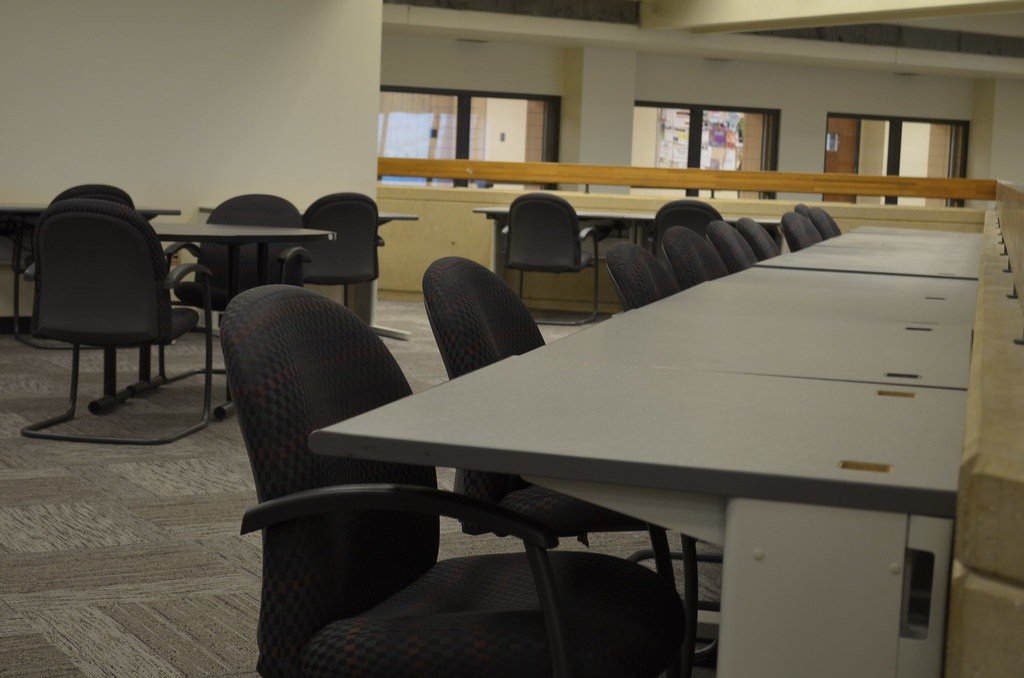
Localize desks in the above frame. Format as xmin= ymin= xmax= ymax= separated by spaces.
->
xmin=91 ymin=221 xmax=338 ymax=420
xmin=0 ymin=196 xmax=184 ymax=223
xmin=316 ymin=231 xmax=984 ymax=678
xmin=199 ymin=203 xmax=421 ymax=345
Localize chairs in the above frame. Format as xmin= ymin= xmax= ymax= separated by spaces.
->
xmin=497 ymin=191 xmax=602 ymax=327
xmin=652 ymin=198 xmax=725 ymax=260
xmin=736 ymin=215 xmax=780 ymax=259
xmin=708 ymin=220 xmax=758 ymax=269
xmin=662 ymin=223 xmax=729 ymax=285
xmin=420 ymin=251 xmax=722 ymax=660
xmin=778 ymin=211 xmax=823 ymax=251
xmin=300 ymin=189 xmax=394 ymax=290
xmin=163 ymin=190 xmax=317 ymax=403
xmin=20 ymin=198 xmax=216 ymax=452
xmin=791 ymin=202 xmax=811 ymax=218
xmin=598 ymin=239 xmax=679 ymax=309
xmin=812 ymin=206 xmax=842 ymax=240
xmin=19 ymin=182 xmax=137 ymax=354
xmin=213 ymin=280 xmax=698 ymax=678
